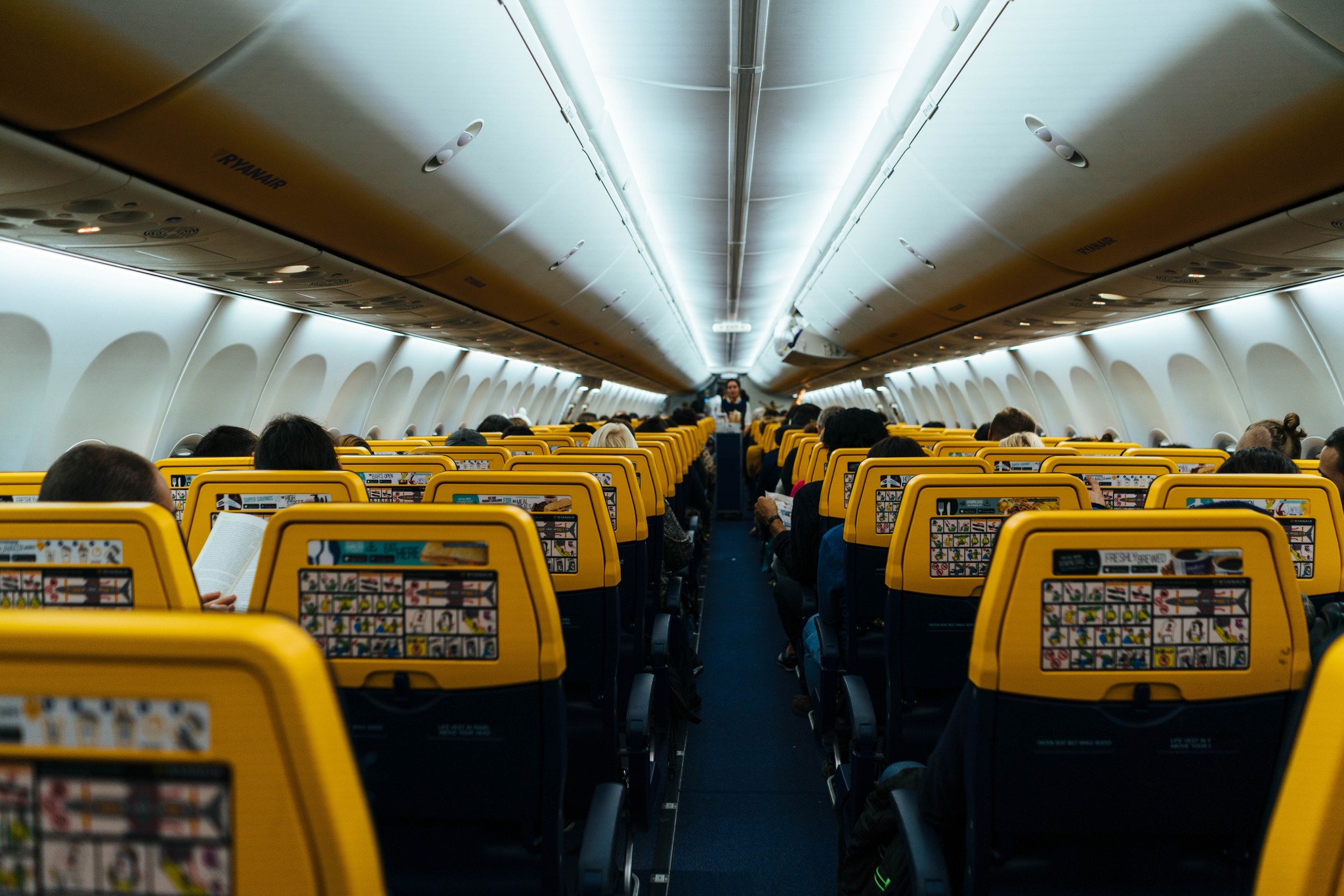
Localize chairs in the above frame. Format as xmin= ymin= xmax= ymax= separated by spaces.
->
xmin=0 ymin=409 xmax=1344 ymax=896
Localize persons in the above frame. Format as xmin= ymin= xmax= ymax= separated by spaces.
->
xmin=37 ymin=444 xmax=237 ymax=611
xmin=720 ymin=379 xmax=752 ymax=438
xmin=194 ymin=425 xmax=259 ymax=457
xmin=444 ymin=428 xmax=488 ymax=446
xmin=335 ymin=434 xmax=372 ymax=455
xmin=1215 ymin=413 xmax=1344 ymax=512
xmin=755 ymin=400 xmax=1046 ymax=668
xmin=254 ymin=412 xmax=343 ymax=471
xmin=476 ymin=398 xmax=717 ymax=571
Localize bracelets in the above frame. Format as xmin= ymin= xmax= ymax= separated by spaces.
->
xmin=767 ymin=515 xmax=784 ymax=528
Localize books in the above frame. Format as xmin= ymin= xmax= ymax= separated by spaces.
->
xmin=192 ymin=512 xmax=268 ymax=612
